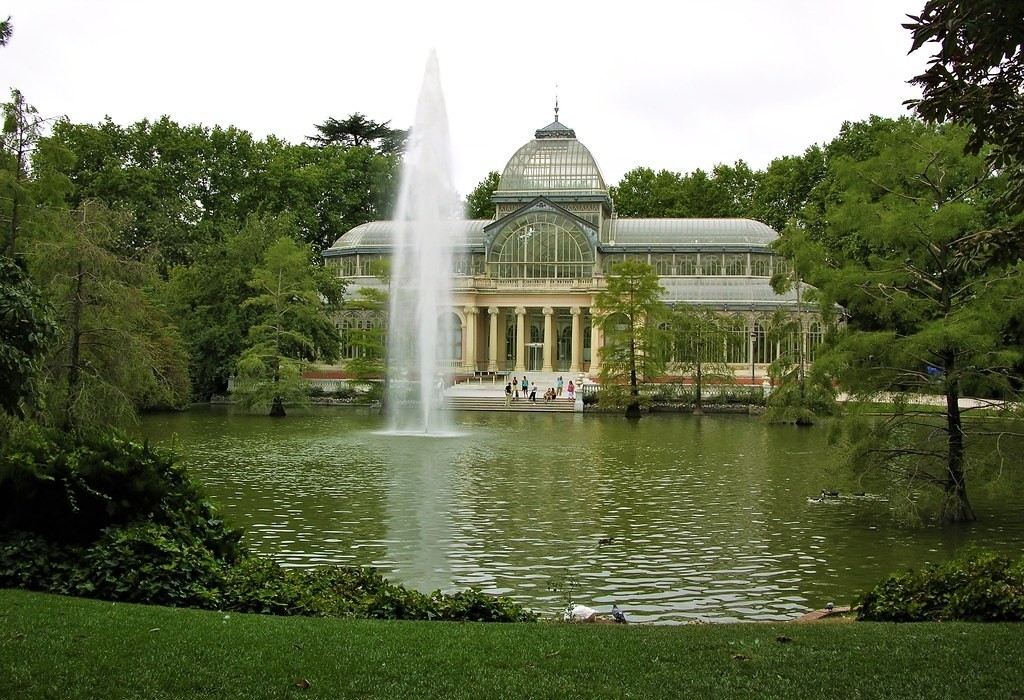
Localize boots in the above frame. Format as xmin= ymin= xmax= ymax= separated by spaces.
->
xmin=514 ymin=397 xmax=518 ymax=401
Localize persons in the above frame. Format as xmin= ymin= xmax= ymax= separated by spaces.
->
xmin=511 ymin=377 xmax=519 ymax=398
xmin=526 ymin=382 xmax=537 ymax=405
xmin=543 ymin=375 xmax=563 ymax=403
xmin=521 ymin=375 xmax=529 ymax=397
xmin=504 ymin=382 xmax=512 ymax=407
xmin=514 ymin=389 xmax=519 ymax=400
xmin=437 ymin=378 xmax=446 ymax=409
xmin=567 ymin=381 xmax=574 ymax=401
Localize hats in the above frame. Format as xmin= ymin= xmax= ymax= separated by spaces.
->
xmin=523 ymin=376 xmax=527 ymax=378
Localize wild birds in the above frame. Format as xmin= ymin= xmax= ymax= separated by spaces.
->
xmin=805 ymin=488 xmax=867 ymax=504
xmin=777 ymin=636 xmax=792 ymax=644
xmin=732 ymin=654 xmax=752 ymax=661
xmin=611 ymin=603 xmax=626 ymax=624
xmin=825 ymin=601 xmax=836 ymax=612
xmin=598 ymin=537 xmax=614 ymax=543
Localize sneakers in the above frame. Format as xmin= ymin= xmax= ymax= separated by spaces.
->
xmin=532 ymin=404 xmax=537 ymax=405
xmin=525 ymin=400 xmax=529 ymax=402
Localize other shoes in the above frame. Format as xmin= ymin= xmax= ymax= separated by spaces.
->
xmin=504 ymin=405 xmax=512 ymax=408
